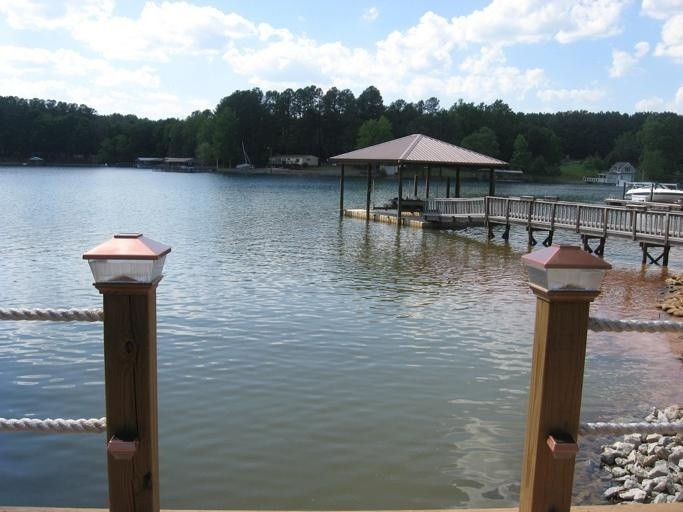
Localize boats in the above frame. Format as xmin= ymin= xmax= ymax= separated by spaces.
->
xmin=624 ymin=188 xmax=683 ymax=203
